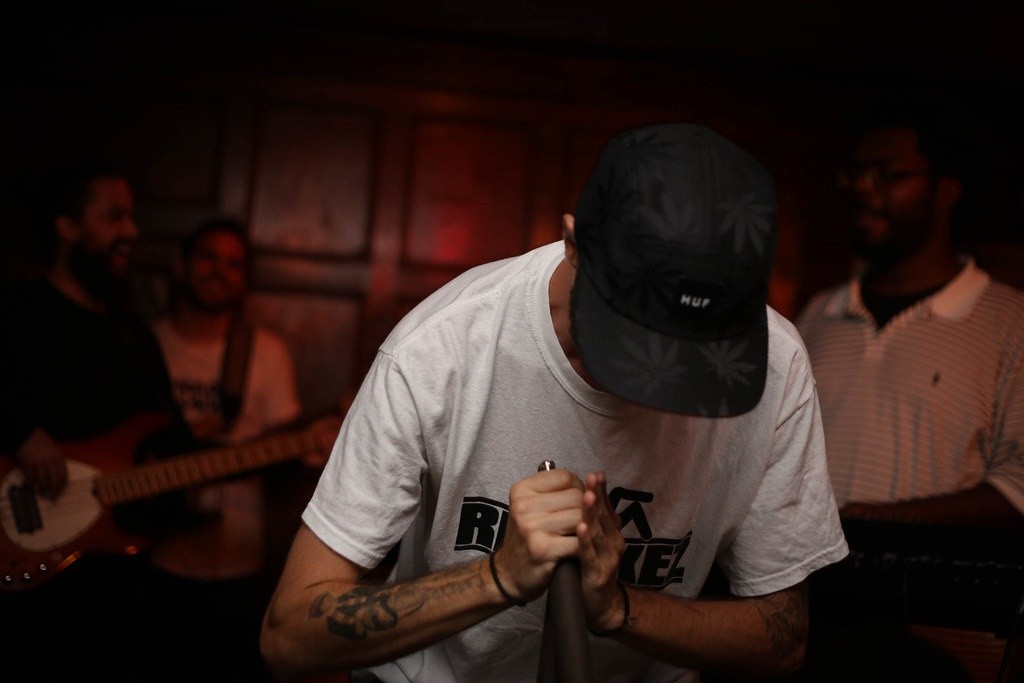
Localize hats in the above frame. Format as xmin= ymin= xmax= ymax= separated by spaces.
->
xmin=570 ymin=122 xmax=783 ymax=420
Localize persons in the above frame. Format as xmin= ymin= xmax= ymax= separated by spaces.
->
xmin=0 ymin=157 xmax=226 ymax=683
xmin=144 ymin=213 xmax=307 ymax=682
xmin=255 ymin=126 xmax=850 ymax=683
xmin=793 ymin=111 xmax=1024 ymax=683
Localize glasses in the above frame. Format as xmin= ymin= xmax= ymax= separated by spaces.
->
xmin=849 ymin=162 xmax=930 ymax=184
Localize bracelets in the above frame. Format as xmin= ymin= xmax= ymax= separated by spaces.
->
xmin=587 ymin=580 xmax=630 ymax=640
xmin=487 ymin=549 xmax=528 ymax=609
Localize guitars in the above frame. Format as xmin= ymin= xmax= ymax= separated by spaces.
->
xmin=0 ymin=403 xmax=351 ymax=593
